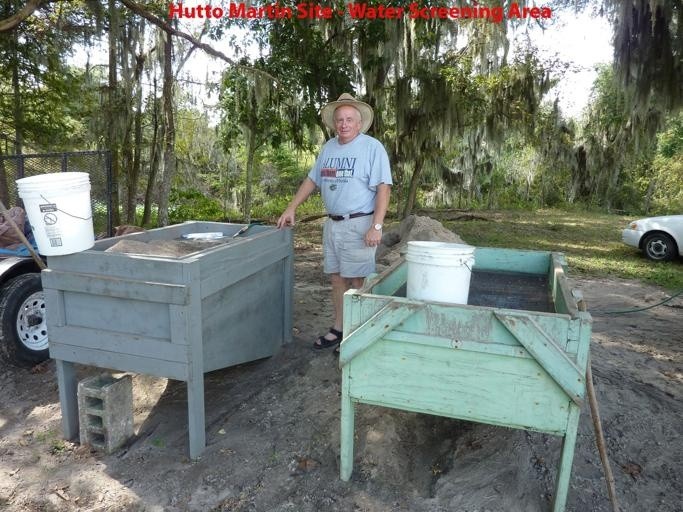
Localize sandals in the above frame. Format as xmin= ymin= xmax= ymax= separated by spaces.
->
xmin=313 ymin=329 xmax=343 ymax=349
xmin=333 ymin=345 xmax=340 ymax=355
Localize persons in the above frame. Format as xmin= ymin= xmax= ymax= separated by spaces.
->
xmin=275 ymin=92 xmax=396 ymax=358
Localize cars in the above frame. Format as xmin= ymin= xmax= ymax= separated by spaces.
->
xmin=0 ymin=248 xmax=51 ymax=368
xmin=621 ymin=213 xmax=683 ymax=262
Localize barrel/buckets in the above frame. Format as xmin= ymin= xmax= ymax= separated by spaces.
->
xmin=14 ymin=170 xmax=96 ymax=257
xmin=404 ymin=241 xmax=476 ymax=306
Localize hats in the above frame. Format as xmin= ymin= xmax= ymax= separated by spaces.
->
xmin=321 ymin=93 xmax=374 ymax=134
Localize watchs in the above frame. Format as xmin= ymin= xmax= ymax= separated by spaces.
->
xmin=371 ymin=222 xmax=383 ymax=230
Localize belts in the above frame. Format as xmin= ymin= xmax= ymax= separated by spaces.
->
xmin=328 ymin=211 xmax=374 ymax=221
